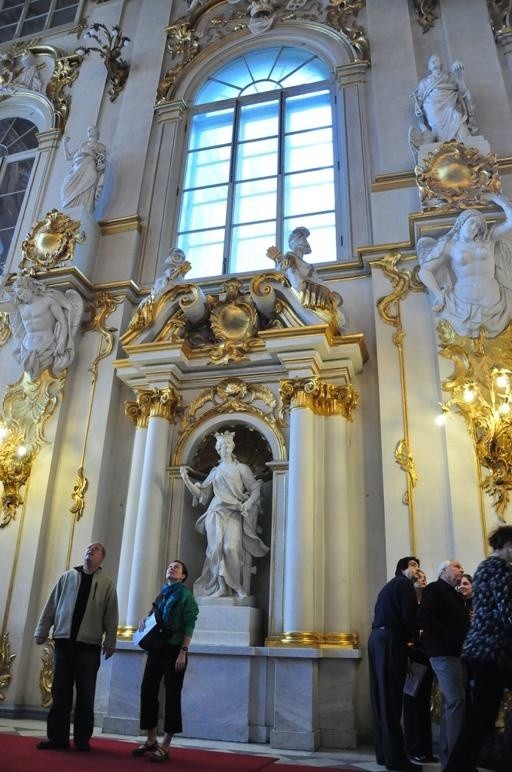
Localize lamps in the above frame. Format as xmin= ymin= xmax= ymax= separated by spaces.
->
xmin=0 ymin=429 xmax=36 ymax=530
xmin=75 ymin=23 xmax=132 ymax=103
xmin=460 ymin=363 xmax=512 ymax=524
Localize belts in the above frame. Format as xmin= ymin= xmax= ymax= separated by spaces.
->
xmin=372 ymin=625 xmax=397 ymax=631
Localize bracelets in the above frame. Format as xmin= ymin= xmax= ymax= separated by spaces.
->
xmin=179 ymin=645 xmax=189 ymax=652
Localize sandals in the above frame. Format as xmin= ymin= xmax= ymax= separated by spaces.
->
xmin=149 ymin=746 xmax=172 ymax=762
xmin=129 ymin=738 xmax=160 ymax=757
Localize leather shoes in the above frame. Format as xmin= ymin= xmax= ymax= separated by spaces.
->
xmin=35 ymin=735 xmax=92 ymax=751
xmin=408 ymin=747 xmax=438 ymax=764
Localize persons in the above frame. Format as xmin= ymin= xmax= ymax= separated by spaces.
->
xmin=131 ymin=560 xmax=198 ymax=762
xmin=32 ymin=540 xmax=119 ymax=753
xmin=368 ymin=525 xmax=512 ymax=772
xmin=8 ymin=275 xmax=82 ymax=381
xmin=57 ymin=125 xmax=111 ymax=215
xmin=408 ymin=50 xmax=479 ymax=143
xmin=177 ymin=427 xmax=266 ymax=601
xmin=415 ymin=193 xmax=511 ymax=338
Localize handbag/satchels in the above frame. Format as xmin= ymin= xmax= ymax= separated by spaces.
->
xmin=139 ymin=605 xmax=174 ymax=655
